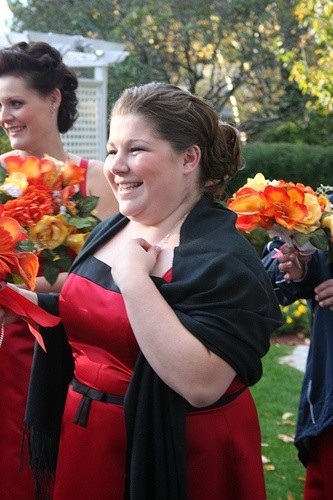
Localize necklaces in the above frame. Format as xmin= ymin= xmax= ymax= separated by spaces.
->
xmin=16 ymin=150 xmax=20 ymax=157
xmin=158 ymin=212 xmax=190 ymax=247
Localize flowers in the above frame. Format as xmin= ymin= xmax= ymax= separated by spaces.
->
xmin=226 ymin=173 xmax=333 ymax=269
xmin=0 ymin=152 xmax=103 ymax=292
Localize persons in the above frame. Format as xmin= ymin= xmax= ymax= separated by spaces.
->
xmin=261 ymin=194 xmax=333 ymax=500
xmin=0 ymin=81 xmax=285 ymax=500
xmin=0 ymin=41 xmax=119 ymax=500
